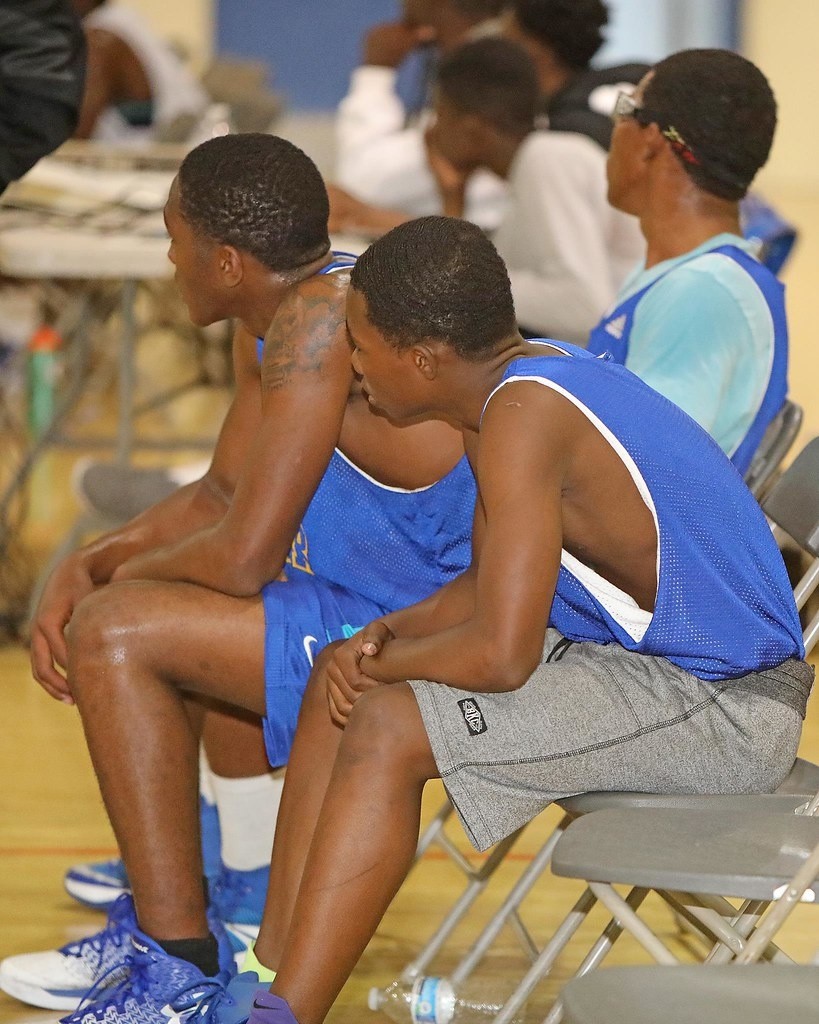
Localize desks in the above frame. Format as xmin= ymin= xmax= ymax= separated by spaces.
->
xmin=0 ymin=135 xmax=189 ymax=646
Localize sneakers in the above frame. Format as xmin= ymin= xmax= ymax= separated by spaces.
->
xmin=65 ymin=792 xmax=271 ymax=939
xmin=58 ymin=929 xmax=238 ymax=1024
xmin=170 ymin=971 xmax=275 ymax=1024
xmin=0 ymin=892 xmax=238 ymax=1012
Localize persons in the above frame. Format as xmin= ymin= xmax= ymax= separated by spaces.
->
xmin=165 ymin=213 xmax=816 ymax=1024
xmin=425 ymin=33 xmax=648 ymax=341
xmin=1 ymin=0 xmax=652 ymax=256
xmin=0 ymin=129 xmax=481 ymax=1024
xmin=65 ymin=44 xmax=794 ymax=955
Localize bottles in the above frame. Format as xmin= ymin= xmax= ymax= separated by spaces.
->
xmin=369 ymin=974 xmax=527 ymax=1024
xmin=25 ymin=324 xmax=64 ymax=443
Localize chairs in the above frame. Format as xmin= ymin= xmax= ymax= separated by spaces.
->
xmin=57 ymin=60 xmax=286 ymax=376
xmin=397 ymin=395 xmax=819 ymax=1024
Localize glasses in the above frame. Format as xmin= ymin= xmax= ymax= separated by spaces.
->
xmin=613 ymin=91 xmax=649 ymax=126
